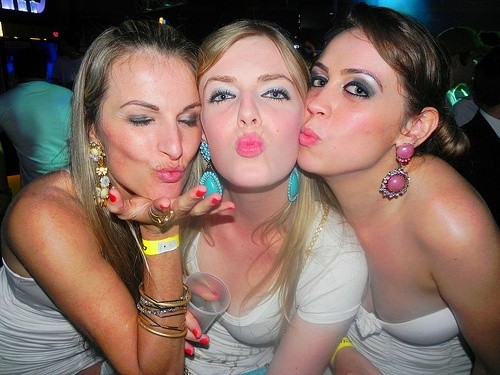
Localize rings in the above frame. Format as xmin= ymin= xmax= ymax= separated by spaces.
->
xmin=149 ymin=207 xmax=174 ymax=226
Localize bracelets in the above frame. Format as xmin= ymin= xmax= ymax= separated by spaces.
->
xmin=142 ymin=234 xmax=179 ymax=256
xmin=329 ymin=338 xmax=353 ymax=369
xmin=137 ymin=282 xmax=190 ymax=338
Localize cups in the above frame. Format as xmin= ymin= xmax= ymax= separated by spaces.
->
xmin=184 ymin=271 xmax=231 ymax=332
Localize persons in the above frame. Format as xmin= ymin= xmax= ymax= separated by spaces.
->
xmin=177 ymin=21 xmax=369 ymax=375
xmin=436 ymin=27 xmax=500 ymax=232
xmin=296 ymin=0 xmax=500 ymax=375
xmin=0 ymin=40 xmax=74 ymax=188
xmin=0 ymin=18 xmax=235 ymax=375
